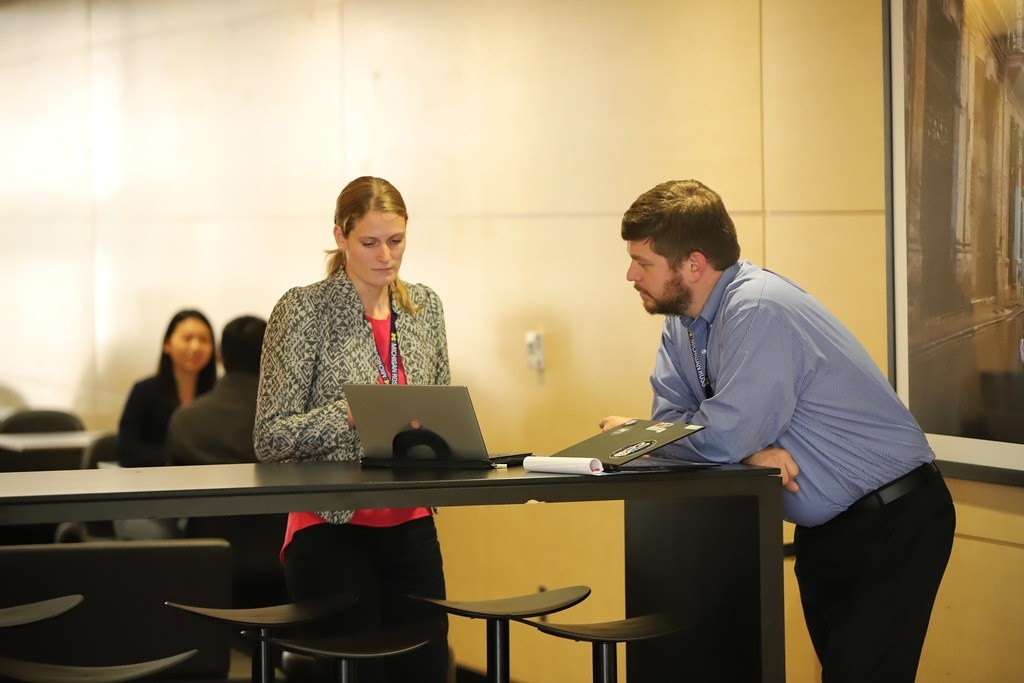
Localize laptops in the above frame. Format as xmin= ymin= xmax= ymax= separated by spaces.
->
xmin=547 ymin=419 xmax=723 ymax=473
xmin=342 ymin=383 xmax=534 ymax=462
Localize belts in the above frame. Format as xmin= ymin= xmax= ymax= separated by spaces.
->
xmin=822 ymin=461 xmax=939 ymax=528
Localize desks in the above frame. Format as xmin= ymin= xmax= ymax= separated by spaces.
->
xmin=0 ymin=427 xmax=118 ymax=468
xmin=0 ymin=463 xmax=786 ymax=683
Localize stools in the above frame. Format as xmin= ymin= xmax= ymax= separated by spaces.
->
xmin=0 ymin=586 xmax=693 ymax=683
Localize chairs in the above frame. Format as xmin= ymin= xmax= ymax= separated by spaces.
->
xmin=0 ymin=410 xmax=86 ymax=472
xmin=79 ymin=434 xmax=121 ymax=539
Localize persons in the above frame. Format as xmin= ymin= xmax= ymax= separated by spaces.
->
xmin=164 ymin=317 xmax=292 ymax=606
xmin=597 ymin=180 xmax=958 ymax=683
xmin=252 ymin=176 xmax=459 ymax=683
xmin=117 ymin=310 xmax=248 ymax=539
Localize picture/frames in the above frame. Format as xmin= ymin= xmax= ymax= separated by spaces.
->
xmin=880 ymin=0 xmax=1024 ymax=487
